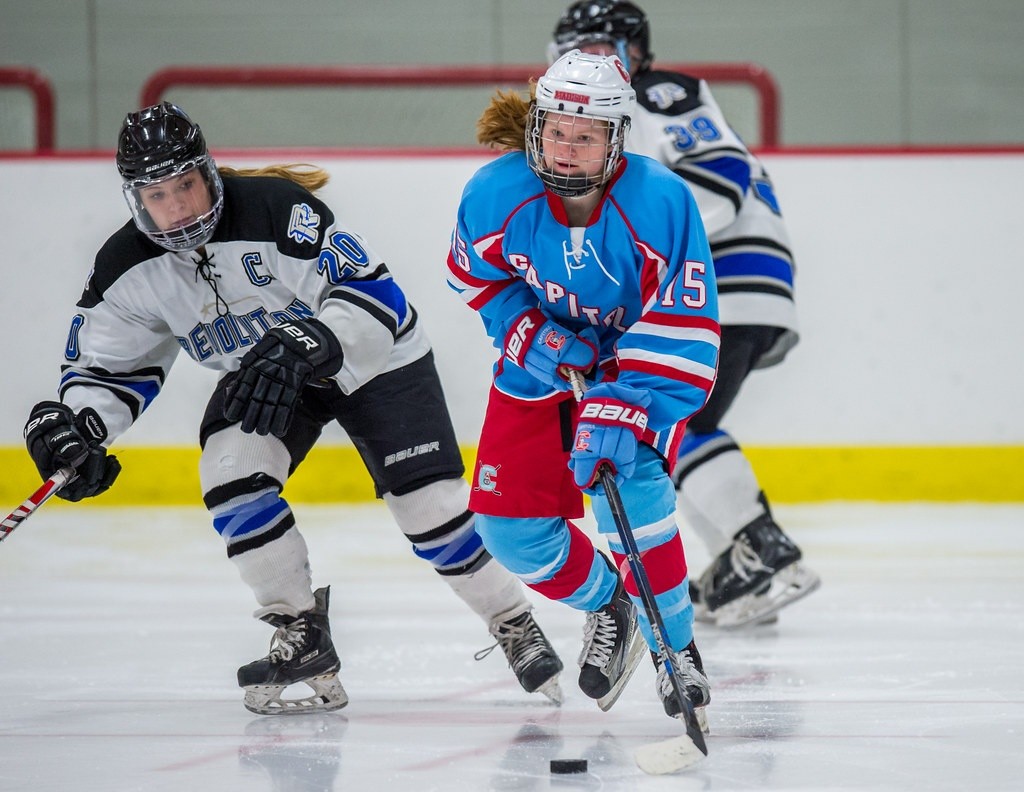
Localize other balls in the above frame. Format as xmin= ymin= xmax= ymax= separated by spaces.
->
xmin=550 ymin=758 xmax=588 ymax=774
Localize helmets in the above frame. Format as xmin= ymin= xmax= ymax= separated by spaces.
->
xmin=545 ymin=0 xmax=653 ymax=88
xmin=524 ymin=48 xmax=636 ymax=199
xmin=114 ymin=101 xmax=223 ymax=252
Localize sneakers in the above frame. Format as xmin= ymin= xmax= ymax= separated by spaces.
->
xmin=707 ymin=489 xmax=821 ymax=625
xmin=576 ymin=550 xmax=649 ymax=711
xmin=650 ymin=636 xmax=711 ymax=737
xmin=237 ymin=585 xmax=349 ymax=714
xmin=473 ymin=602 xmax=563 ymax=706
xmin=686 ymin=547 xmax=778 ymax=626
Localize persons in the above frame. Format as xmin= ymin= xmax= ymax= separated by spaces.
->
xmin=25 ymin=102 xmax=563 ymax=715
xmin=448 ymin=49 xmax=711 ymax=732
xmin=550 ymin=0 xmax=822 ymax=629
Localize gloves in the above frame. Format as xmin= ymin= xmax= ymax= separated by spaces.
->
xmin=493 ymin=306 xmax=598 ymax=392
xmin=24 ymin=401 xmax=122 ymax=503
xmin=568 ymin=382 xmax=652 ymax=496
xmin=223 ymin=316 xmax=344 ymax=439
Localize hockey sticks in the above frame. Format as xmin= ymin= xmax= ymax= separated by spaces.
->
xmin=0 ymin=462 xmax=85 ymax=541
xmin=571 ymin=365 xmax=710 ymax=777
